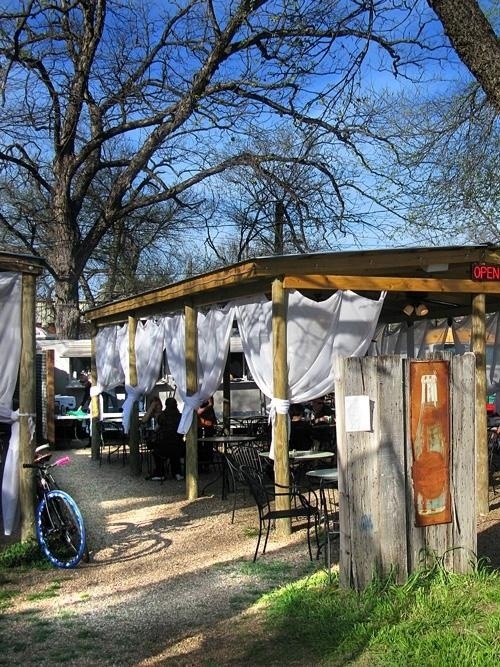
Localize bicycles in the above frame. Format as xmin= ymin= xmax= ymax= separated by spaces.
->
xmin=23 ymin=451 xmax=89 ymax=568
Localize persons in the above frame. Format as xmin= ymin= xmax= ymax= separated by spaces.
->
xmin=77 ymin=375 xmax=92 ymax=449
xmin=139 ymin=396 xmax=217 ymax=482
xmin=290 ymin=399 xmax=336 ymax=486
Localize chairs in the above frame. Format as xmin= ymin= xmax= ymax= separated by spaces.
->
xmin=95 ymin=415 xmax=342 ymax=574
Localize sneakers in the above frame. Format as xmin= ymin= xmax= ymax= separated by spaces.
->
xmin=152 ymin=474 xmax=165 ymax=480
xmin=176 ymin=473 xmax=184 ymax=480
